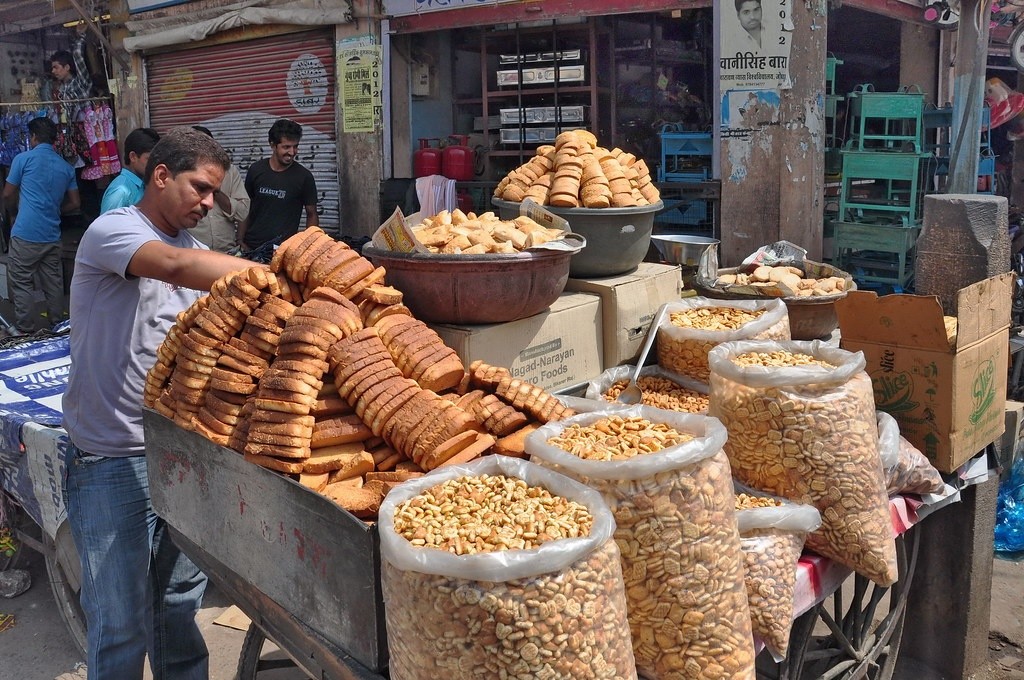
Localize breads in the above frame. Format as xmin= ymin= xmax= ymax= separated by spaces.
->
xmin=718 ymin=265 xmax=844 ymax=298
xmin=141 ymin=226 xmax=579 ymax=520
xmin=407 ymin=208 xmax=566 ymax=256
xmin=492 ymin=129 xmax=661 ymax=208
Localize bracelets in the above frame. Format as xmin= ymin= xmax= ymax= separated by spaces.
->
xmin=237 ymin=239 xmax=244 ymax=241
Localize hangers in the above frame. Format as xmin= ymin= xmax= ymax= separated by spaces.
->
xmin=5 ymin=96 xmax=107 ymax=118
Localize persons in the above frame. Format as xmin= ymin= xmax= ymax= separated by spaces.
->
xmin=724 ymin=0 xmax=771 ymax=49
xmin=237 ymin=119 xmax=319 ymax=252
xmin=100 ymin=128 xmax=160 ymax=215
xmin=50 ymin=20 xmax=92 ymax=169
xmin=61 ymin=126 xmax=272 ymax=680
xmin=185 ymin=125 xmax=251 ymax=251
xmin=0 ymin=117 xmax=78 ymax=333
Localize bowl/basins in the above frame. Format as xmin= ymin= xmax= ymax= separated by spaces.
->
xmin=362 ymin=230 xmax=572 ymax=326
xmin=490 ymin=195 xmax=662 ymax=276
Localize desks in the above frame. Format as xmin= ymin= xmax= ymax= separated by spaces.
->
xmin=658 ymin=132 xmax=713 ymax=181
xmin=823 ymin=49 xmax=997 ymax=294
xmin=654 ymin=165 xmax=707 ymax=225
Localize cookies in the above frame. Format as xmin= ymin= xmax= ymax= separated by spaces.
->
xmin=379 ymin=306 xmax=945 ymax=680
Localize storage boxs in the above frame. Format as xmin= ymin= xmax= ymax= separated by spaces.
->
xmin=426 ymin=289 xmax=603 ymax=393
xmin=835 ymin=272 xmax=1016 ymax=476
xmin=561 ymin=261 xmax=683 ymax=372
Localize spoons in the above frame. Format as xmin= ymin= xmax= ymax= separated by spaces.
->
xmin=616 ymin=302 xmax=667 ymax=406
